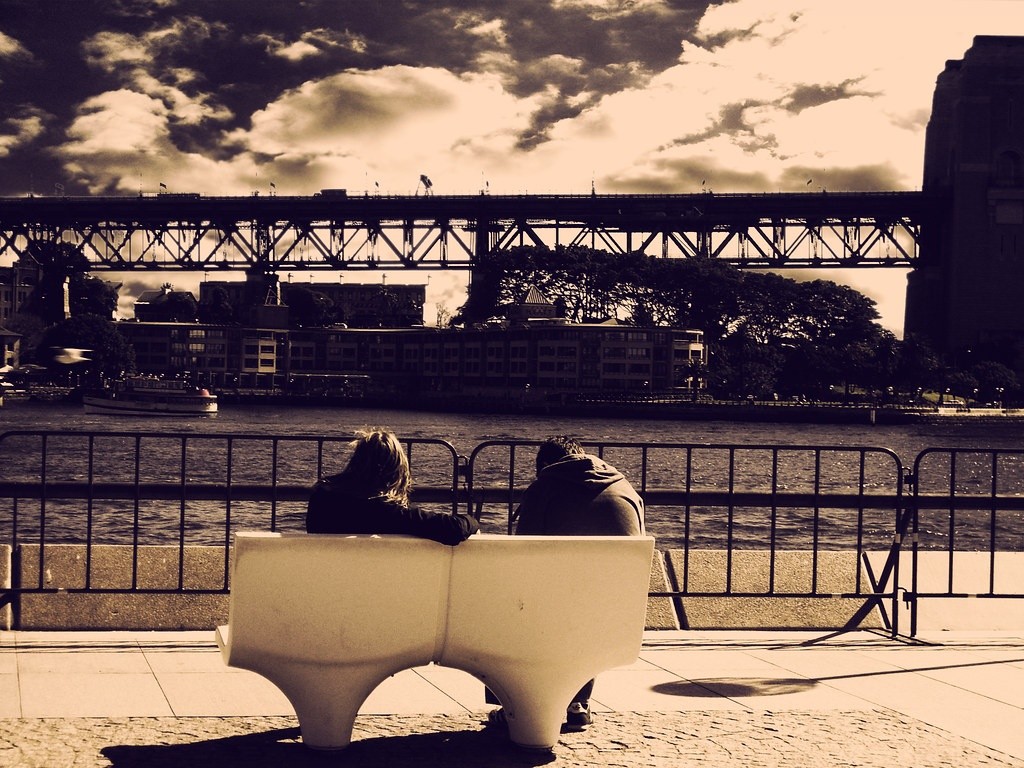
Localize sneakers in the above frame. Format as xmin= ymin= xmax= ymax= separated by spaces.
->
xmin=488 ymin=707 xmax=508 ymax=729
xmin=567 ymin=702 xmax=593 ymax=724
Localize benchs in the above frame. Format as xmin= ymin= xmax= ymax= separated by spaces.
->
xmin=214 ymin=530 xmax=656 ymax=752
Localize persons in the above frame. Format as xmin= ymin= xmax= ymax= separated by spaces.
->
xmin=480 ymin=434 xmax=646 ymax=732
xmin=306 ymin=425 xmax=480 ymax=546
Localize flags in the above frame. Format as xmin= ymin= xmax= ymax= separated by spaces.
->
xmin=270 ymin=182 xmax=275 ymax=188
xmin=420 ymin=174 xmax=433 ymax=189
xmin=160 ymin=183 xmax=166 ymax=188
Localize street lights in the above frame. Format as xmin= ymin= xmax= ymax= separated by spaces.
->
xmin=916 ymin=387 xmax=922 ymax=407
xmin=996 ymin=387 xmax=1004 ymax=408
xmin=946 ymin=387 xmax=951 ymax=407
xmin=888 ymin=386 xmax=894 ymax=404
xmin=974 ymin=388 xmax=980 ymax=408
xmin=643 ymin=380 xmax=648 ymax=397
xmin=829 ymin=384 xmax=834 ymax=402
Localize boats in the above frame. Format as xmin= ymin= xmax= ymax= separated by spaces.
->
xmin=82 ymin=371 xmax=218 ymax=418
